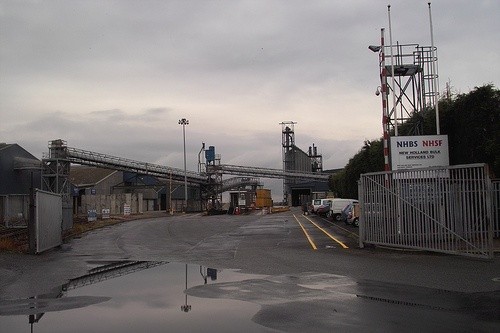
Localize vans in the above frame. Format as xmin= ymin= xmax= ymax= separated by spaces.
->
xmin=311 ymin=200 xmax=332 ymax=216
xmin=329 ymin=200 xmax=359 ymax=222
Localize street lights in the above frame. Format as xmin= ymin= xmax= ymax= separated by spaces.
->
xmin=178 ymin=118 xmax=189 ymax=215
xmin=180 ymin=265 xmax=191 ymax=312
xmin=368 ymin=28 xmax=390 ymax=190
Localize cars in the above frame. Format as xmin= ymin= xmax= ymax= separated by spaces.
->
xmin=347 ymin=204 xmax=384 ymax=228
xmin=341 ymin=202 xmax=359 ymax=225
xmin=317 ymin=204 xmax=329 ymax=219
